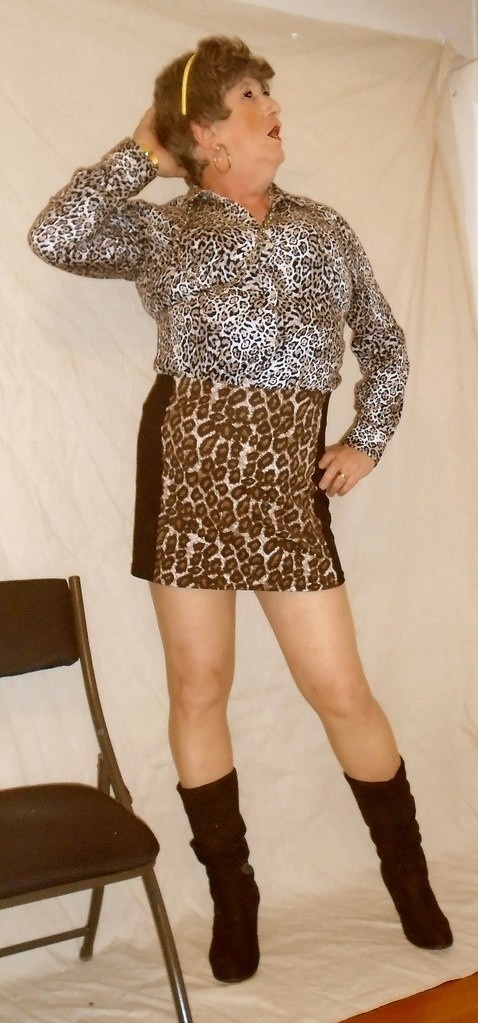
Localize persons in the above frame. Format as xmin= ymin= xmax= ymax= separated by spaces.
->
xmin=28 ymin=37 xmax=452 ymax=983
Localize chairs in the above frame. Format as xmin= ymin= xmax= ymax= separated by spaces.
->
xmin=0 ymin=576 xmax=192 ymax=1023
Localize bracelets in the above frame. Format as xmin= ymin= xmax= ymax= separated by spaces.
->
xmin=135 ymin=140 xmax=160 ymax=168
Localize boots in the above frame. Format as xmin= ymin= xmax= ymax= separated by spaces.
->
xmin=175 ymin=765 xmax=261 ymax=982
xmin=344 ymin=753 xmax=454 ymax=950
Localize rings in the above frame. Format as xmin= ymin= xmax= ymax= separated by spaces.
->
xmin=336 ymin=472 xmax=348 ymax=480
